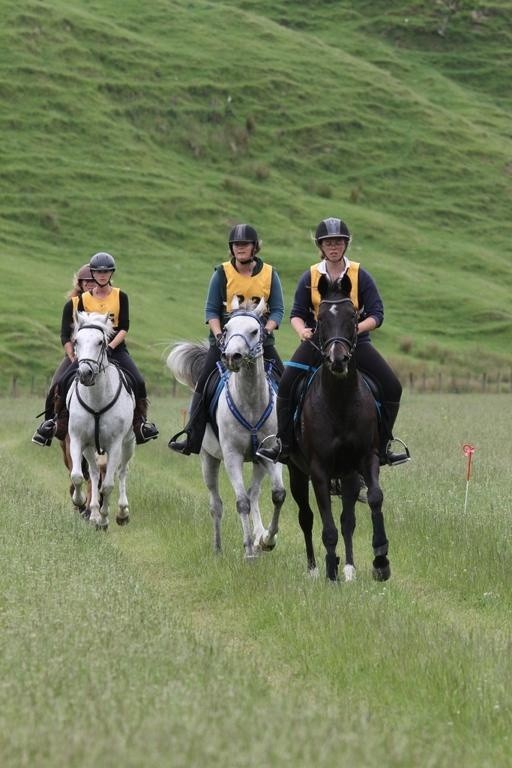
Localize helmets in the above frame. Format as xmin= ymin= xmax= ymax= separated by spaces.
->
xmin=315 ymin=218 xmax=350 ymax=240
xmin=77 ymin=252 xmax=116 ymax=280
xmin=228 ymin=224 xmax=257 ymax=243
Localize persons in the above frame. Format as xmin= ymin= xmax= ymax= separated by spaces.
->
xmin=33 ymin=264 xmax=98 ymax=446
xmin=168 ymin=223 xmax=286 ymax=455
xmin=38 ymin=252 xmax=158 ymax=444
xmin=257 ymin=216 xmax=408 ymax=465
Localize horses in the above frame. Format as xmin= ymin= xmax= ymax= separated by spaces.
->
xmin=286 ymin=271 xmax=394 ymax=585
xmin=56 ymin=312 xmax=140 ymax=532
xmin=162 ymin=293 xmax=288 ymax=561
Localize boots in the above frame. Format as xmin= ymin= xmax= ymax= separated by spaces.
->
xmin=133 ymin=397 xmax=159 ymax=445
xmin=34 ymin=392 xmax=69 ymax=446
xmin=170 ymin=392 xmax=206 ymax=454
xmin=258 ymin=397 xmax=294 ymax=464
xmin=379 ymin=402 xmax=408 ymax=466
